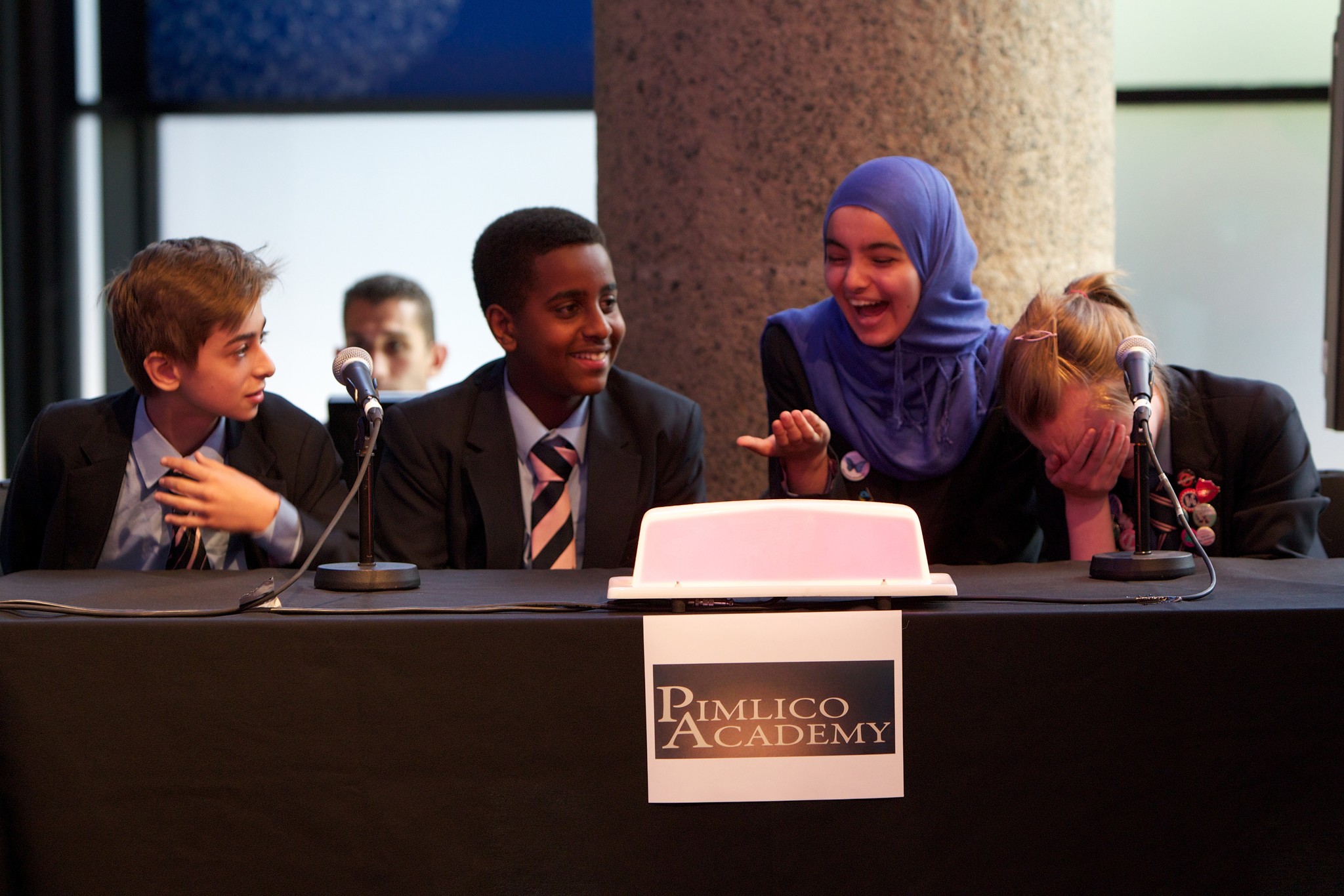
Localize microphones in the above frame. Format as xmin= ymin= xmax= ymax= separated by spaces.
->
xmin=1115 ymin=335 xmax=1156 ymax=422
xmin=333 ymin=348 xmax=385 ymax=421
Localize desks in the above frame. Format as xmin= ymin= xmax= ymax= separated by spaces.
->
xmin=0 ymin=559 xmax=1344 ymax=896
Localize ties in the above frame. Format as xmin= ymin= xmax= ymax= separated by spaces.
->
xmin=1145 ymin=467 xmax=1181 ymax=552
xmin=164 ymin=466 xmax=211 ymax=570
xmin=530 ymin=434 xmax=577 ymax=570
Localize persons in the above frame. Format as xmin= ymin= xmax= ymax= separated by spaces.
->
xmin=372 ymin=206 xmax=704 ymax=569
xmin=738 ymin=157 xmax=1049 ymax=563
xmin=1 ymin=236 xmax=390 ymax=574
xmin=321 ymin=273 xmax=447 ymax=429
xmin=997 ymin=262 xmax=1331 ymax=562
